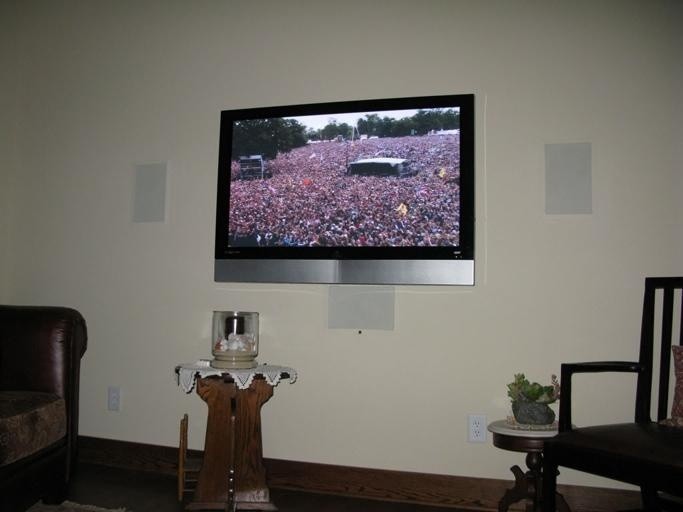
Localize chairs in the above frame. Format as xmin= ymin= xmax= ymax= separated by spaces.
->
xmin=535 ymin=274 xmax=683 ymax=511
xmin=177 ymin=411 xmax=204 ymax=502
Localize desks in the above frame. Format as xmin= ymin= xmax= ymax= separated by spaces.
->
xmin=174 ymin=356 xmax=295 ymax=511
xmin=485 ymin=413 xmax=578 ymax=511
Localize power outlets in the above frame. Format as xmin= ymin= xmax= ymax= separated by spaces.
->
xmin=463 ymin=413 xmax=487 ymax=446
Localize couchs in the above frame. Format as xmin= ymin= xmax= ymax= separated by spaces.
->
xmin=0 ymin=303 xmax=87 ymax=505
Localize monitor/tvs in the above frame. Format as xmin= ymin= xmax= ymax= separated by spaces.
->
xmin=214 ymin=94 xmax=474 ymax=285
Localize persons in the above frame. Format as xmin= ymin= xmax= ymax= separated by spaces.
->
xmin=225 ymin=130 xmax=459 ymax=246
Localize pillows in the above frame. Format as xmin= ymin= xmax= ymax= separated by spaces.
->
xmin=657 ymin=344 xmax=682 ymax=429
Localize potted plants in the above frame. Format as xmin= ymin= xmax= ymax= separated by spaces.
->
xmin=501 ymin=367 xmax=559 ymax=429
